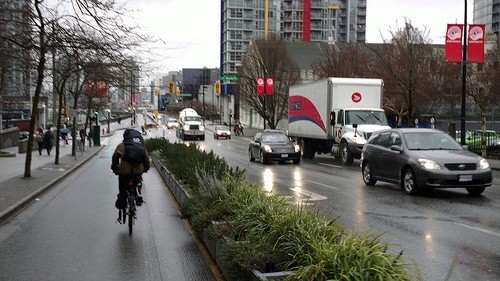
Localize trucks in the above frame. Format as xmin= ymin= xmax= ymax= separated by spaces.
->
xmin=287 ymin=78 xmax=394 ymax=168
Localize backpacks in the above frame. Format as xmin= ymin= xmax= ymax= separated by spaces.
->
xmin=123 ymin=132 xmax=146 ymax=165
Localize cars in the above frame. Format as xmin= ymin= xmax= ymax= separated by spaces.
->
xmin=455 ymin=129 xmax=500 ymax=155
xmin=248 ymin=128 xmax=301 ymax=165
xmin=360 ymin=127 xmax=493 ymax=197
xmin=165 ymin=118 xmax=178 ymax=129
xmin=213 ymin=125 xmax=231 ymax=140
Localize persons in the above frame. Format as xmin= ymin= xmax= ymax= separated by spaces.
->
xmin=44 ymin=126 xmax=55 ymax=154
xmin=110 ymin=129 xmax=150 ymax=224
xmin=233 ymin=119 xmax=243 ymax=135
xmin=60 ymin=132 xmax=67 ymax=139
xmin=35 ymin=130 xmax=44 ymax=155
xmin=80 ymin=128 xmax=87 ymax=142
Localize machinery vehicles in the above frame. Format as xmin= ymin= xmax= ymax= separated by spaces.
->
xmin=175 ymin=107 xmax=205 ymax=141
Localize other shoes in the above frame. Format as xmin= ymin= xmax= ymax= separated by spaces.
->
xmin=136 ymin=196 xmax=143 ymax=206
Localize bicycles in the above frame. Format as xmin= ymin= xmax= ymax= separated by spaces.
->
xmin=111 ymin=166 xmax=147 ymax=235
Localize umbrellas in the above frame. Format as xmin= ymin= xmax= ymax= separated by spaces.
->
xmin=60 ymin=128 xmax=70 ymax=133
